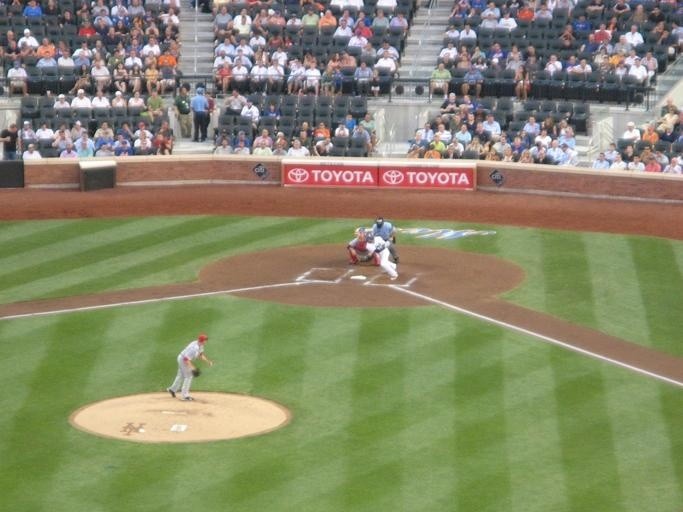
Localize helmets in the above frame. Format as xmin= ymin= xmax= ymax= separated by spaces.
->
xmin=355 ymin=227 xmax=374 ymax=240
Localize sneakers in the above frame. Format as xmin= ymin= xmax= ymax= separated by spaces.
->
xmin=183 ymin=396 xmax=193 ymax=401
xmin=166 ymin=388 xmax=176 ymax=398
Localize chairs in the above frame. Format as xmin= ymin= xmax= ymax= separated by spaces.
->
xmin=2 ymin=0 xmax=682 ymax=163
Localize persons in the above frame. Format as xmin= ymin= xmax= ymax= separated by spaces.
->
xmin=407 ymin=1 xmax=682 ymax=174
xmin=347 ymin=217 xmax=401 ymax=281
xmin=0 ymin=0 xmax=214 ymax=158
xmin=166 ymin=335 xmax=212 ymax=401
xmin=193 ymin=0 xmax=418 ymax=156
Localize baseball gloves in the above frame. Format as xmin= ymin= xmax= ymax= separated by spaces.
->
xmin=359 ymin=255 xmax=373 ymax=262
xmin=192 ymin=368 xmax=201 ymax=376
xmin=374 ymin=244 xmax=385 ymax=252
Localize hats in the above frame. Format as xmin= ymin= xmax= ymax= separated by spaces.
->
xmin=199 ymin=335 xmax=207 ymax=341
xmin=376 ymin=217 xmax=384 ymax=225
